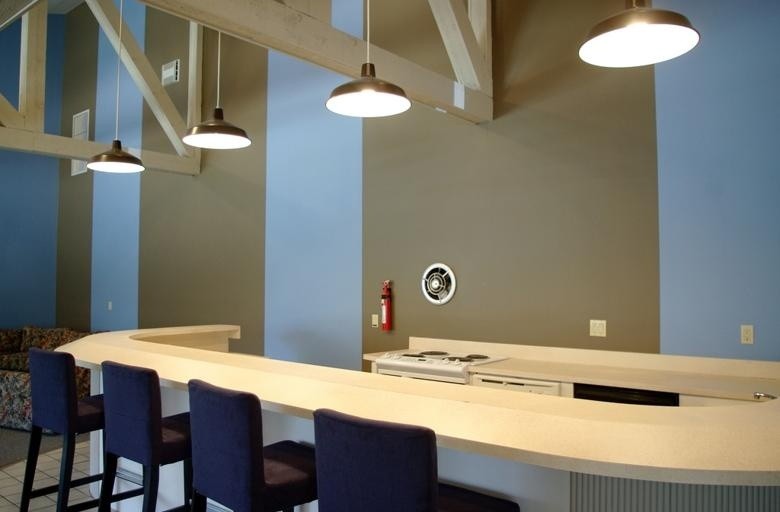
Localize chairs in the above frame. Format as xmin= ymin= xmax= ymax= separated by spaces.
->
xmin=97 ymin=358 xmax=207 ymax=512
xmin=311 ymin=407 xmax=519 ymax=512
xmin=186 ymin=375 xmax=319 ymax=511
xmin=20 ymin=348 xmax=118 ymax=511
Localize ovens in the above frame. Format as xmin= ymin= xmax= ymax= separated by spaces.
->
xmin=377 ymin=363 xmax=466 ymax=384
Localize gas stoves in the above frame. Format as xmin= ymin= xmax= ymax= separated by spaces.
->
xmin=380 ymin=349 xmax=504 ymax=370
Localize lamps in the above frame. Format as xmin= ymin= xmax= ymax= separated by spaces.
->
xmin=85 ymin=2 xmax=146 ymax=177
xmin=325 ymin=1 xmax=412 ymax=122
xmin=183 ymin=28 xmax=252 ymax=152
xmin=578 ymin=0 xmax=701 ymax=73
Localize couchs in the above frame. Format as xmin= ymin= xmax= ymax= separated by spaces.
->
xmin=0 ymin=323 xmax=108 ymax=434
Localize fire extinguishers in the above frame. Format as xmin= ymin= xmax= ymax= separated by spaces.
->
xmin=380 ymin=280 xmax=392 ymax=331
